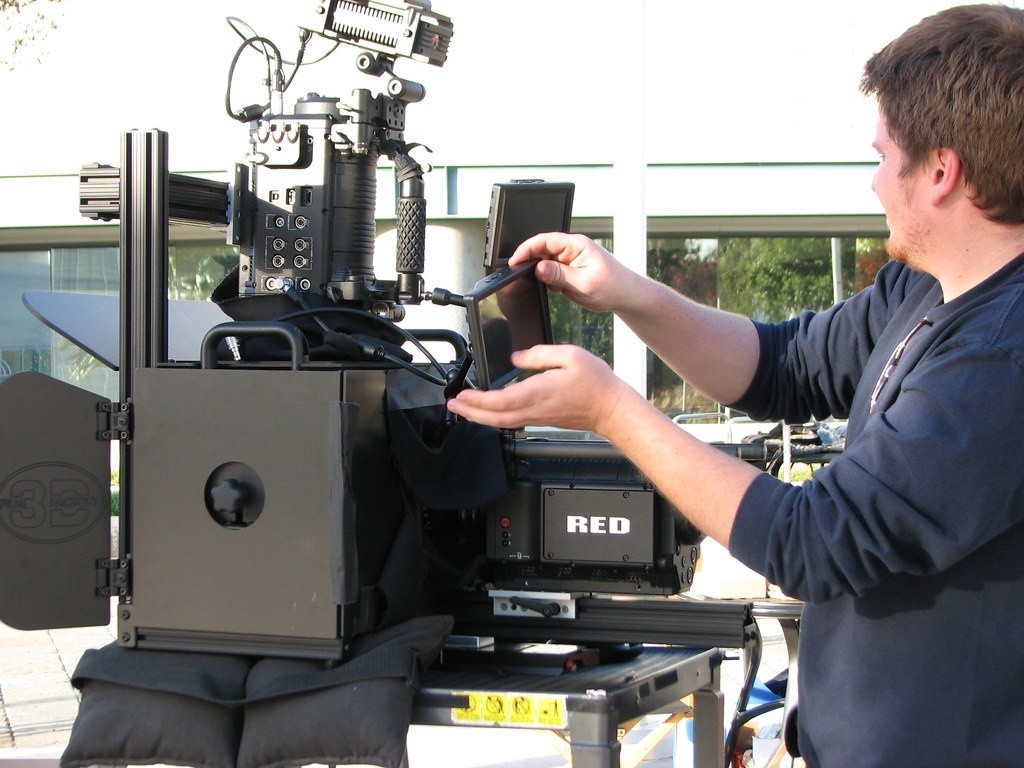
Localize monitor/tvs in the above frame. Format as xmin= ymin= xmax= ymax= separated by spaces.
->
xmin=462 ymin=256 xmax=553 ymax=391
xmin=484 ymin=179 xmax=576 ymax=268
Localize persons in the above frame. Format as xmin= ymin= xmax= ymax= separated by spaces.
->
xmin=447 ymin=1 xmax=1023 ymax=766
